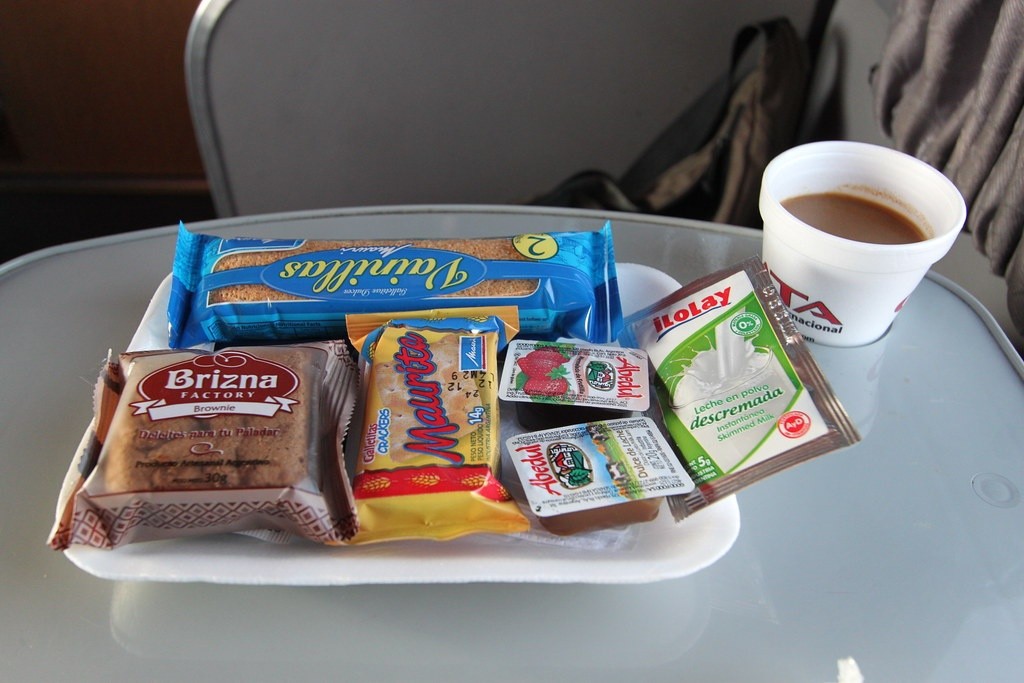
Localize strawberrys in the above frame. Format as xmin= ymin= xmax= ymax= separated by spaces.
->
xmin=515 ymin=346 xmax=572 ymax=397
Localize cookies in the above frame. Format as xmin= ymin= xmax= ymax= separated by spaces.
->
xmin=375 ymin=342 xmax=488 ymax=460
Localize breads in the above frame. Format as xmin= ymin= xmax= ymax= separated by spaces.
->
xmin=208 ymin=235 xmax=539 ymax=304
xmin=106 ymin=350 xmax=313 ymax=496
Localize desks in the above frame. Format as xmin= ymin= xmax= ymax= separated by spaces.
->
xmin=0 ymin=200 xmax=1024 ymax=683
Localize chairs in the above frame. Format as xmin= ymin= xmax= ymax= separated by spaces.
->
xmin=181 ymin=0 xmax=817 ymax=226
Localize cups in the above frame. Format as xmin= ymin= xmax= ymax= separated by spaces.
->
xmin=759 ymin=140 xmax=968 ymax=348
